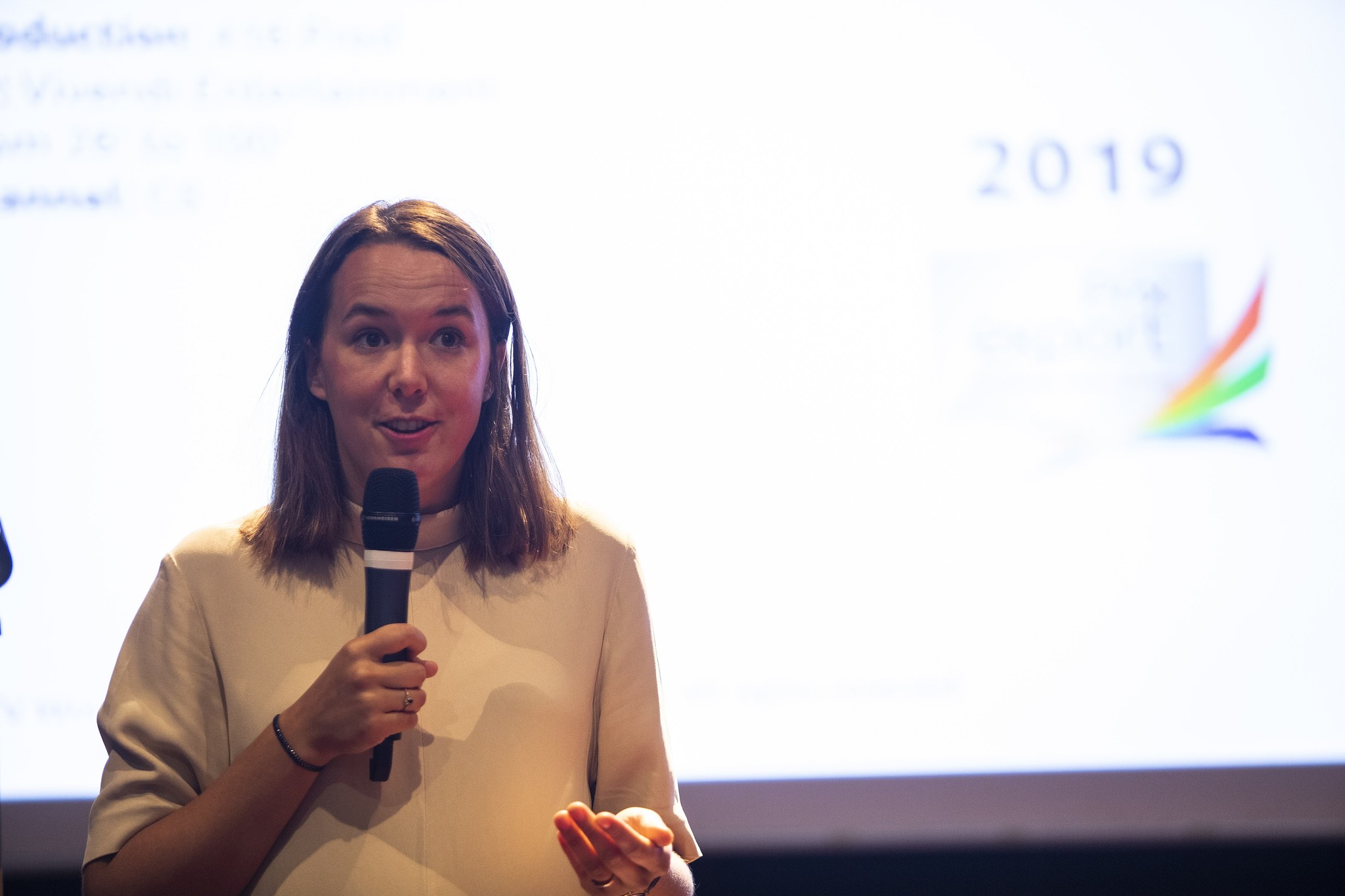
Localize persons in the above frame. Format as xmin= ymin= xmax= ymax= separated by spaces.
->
xmin=75 ymin=197 xmax=702 ymax=896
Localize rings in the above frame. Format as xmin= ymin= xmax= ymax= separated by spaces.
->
xmin=591 ymin=874 xmax=615 ymax=888
xmin=401 ymin=686 xmax=414 ymax=712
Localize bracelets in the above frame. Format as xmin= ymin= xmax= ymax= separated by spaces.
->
xmin=272 ymin=714 xmax=326 ymax=772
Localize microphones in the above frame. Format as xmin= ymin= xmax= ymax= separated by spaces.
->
xmin=359 ymin=467 xmax=422 ymax=783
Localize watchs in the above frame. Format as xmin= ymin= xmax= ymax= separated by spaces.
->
xmin=622 ymin=875 xmax=662 ymax=896
